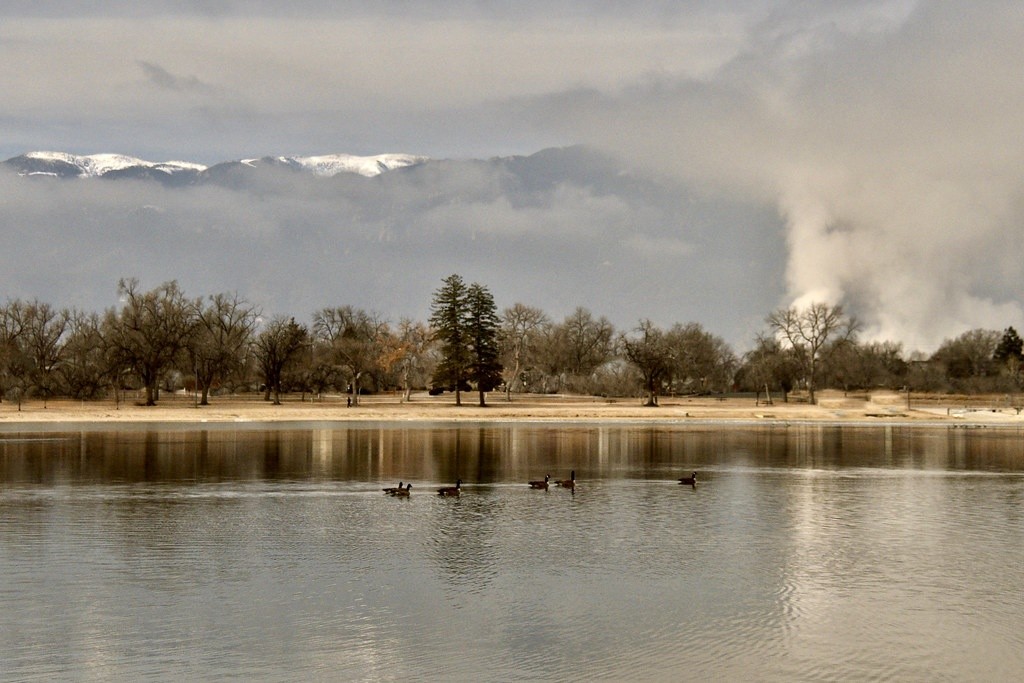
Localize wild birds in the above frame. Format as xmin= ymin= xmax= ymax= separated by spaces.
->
xmin=382 ymin=481 xmax=413 ymax=497
xmin=436 ymin=478 xmax=464 ymax=494
xmin=677 ymin=471 xmax=699 ymax=482
xmin=527 ymin=473 xmax=552 ymax=487
xmin=553 ymin=470 xmax=576 ymax=487
